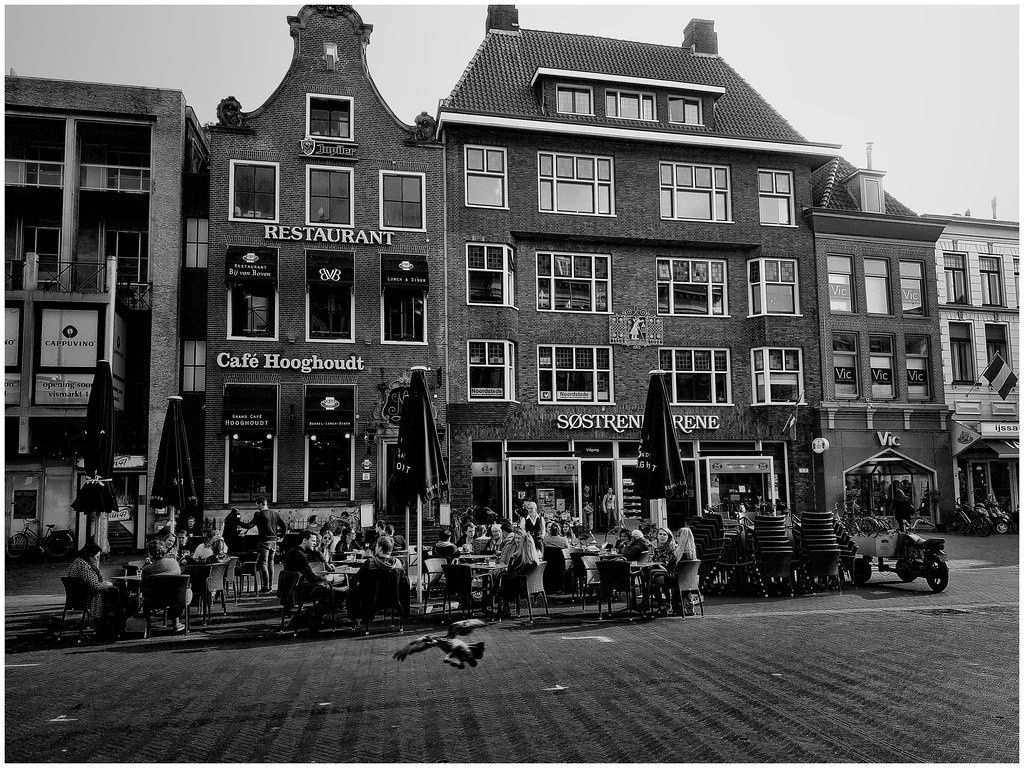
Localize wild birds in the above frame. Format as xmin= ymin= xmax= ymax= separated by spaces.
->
xmin=393 ymin=618 xmax=496 ymax=670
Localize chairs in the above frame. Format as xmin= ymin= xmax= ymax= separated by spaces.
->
xmin=413 ymin=510 xmax=860 ymax=622
xmin=236 ymin=561 xmax=257 ymax=596
xmin=59 ymin=576 xmax=92 ymax=643
xmin=122 ymin=562 xmax=139 ymax=575
xmin=108 ymin=568 xmax=127 ymax=589
xmin=193 ymin=563 xmax=227 ymax=626
xmin=146 ymin=575 xmax=190 ymax=638
xmin=201 ymin=556 xmax=239 ymax=614
xmin=352 ymin=567 xmax=405 ymax=635
xmin=277 ymin=572 xmax=334 ymax=634
xmin=279 ymin=551 xmax=316 ymax=609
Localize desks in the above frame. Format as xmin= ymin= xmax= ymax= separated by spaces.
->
xmin=344 ymin=550 xmax=409 ymax=554
xmin=626 ymin=562 xmax=663 ymax=612
xmin=458 ymin=555 xmax=496 ymax=564
xmin=598 ymin=554 xmax=623 ymax=603
xmin=111 ymin=577 xmax=147 ymax=638
xmin=568 ymin=548 xmax=601 ymax=595
xmin=316 ymin=567 xmax=360 ymax=616
xmin=331 ymin=559 xmax=368 ymax=565
xmin=461 ymin=563 xmax=508 ymax=612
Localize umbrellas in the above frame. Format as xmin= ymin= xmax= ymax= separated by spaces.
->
xmin=71 ymin=359 xmax=119 ymax=571
xmin=150 ymin=396 xmax=198 ymax=535
xmin=393 ymin=365 xmax=450 ymax=603
xmin=632 ymin=369 xmax=688 ymax=530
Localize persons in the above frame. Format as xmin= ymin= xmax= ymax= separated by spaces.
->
xmin=602 ymin=487 xmax=618 ymax=534
xmin=224 ymin=497 xmax=287 ymax=593
xmin=893 ymin=489 xmax=912 ymax=534
xmin=68 ymin=542 xmax=129 ymax=641
xmin=284 ymin=511 xmax=406 ymax=638
xmin=521 ymin=502 xmax=578 ymax=595
xmin=414 ymin=521 xmax=539 ymax=620
xmin=140 ymin=516 xmax=228 ymax=632
xmin=614 ymin=527 xmax=696 ymax=618
xmin=582 ymin=484 xmax=594 ymax=532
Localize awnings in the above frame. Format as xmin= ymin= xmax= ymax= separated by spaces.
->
xmin=221 ymin=384 xmax=355 ymax=438
xmin=224 ymin=246 xmax=430 ymax=297
xmin=981 ymin=438 xmax=1019 ymax=459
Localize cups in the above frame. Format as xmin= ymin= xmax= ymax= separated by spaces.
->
xmin=611 ymin=548 xmax=616 ymax=554
xmin=485 ymin=557 xmax=489 ymax=563
xmin=351 ymin=554 xmax=356 ymax=562
xmin=365 ymin=543 xmax=369 ymax=552
xmin=137 ymin=570 xmax=141 ymax=576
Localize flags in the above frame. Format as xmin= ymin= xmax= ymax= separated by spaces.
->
xmin=983 ymin=352 xmax=1018 ymax=402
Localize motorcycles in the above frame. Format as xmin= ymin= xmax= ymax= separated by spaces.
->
xmin=848 ymin=516 xmax=950 ymax=594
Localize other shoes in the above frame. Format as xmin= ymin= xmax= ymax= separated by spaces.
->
xmin=258 ymin=587 xmax=272 ymax=595
xmin=634 ymin=601 xmax=650 ymax=612
xmin=173 ymin=623 xmax=185 ymax=632
xmin=664 ymin=605 xmax=672 ymax=613
xmin=611 ymin=528 xmax=615 ymax=535
xmin=589 ymin=531 xmax=595 ymax=534
xmin=654 ymin=609 xmax=666 ymax=617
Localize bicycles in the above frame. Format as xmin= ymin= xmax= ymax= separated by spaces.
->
xmin=603 ymin=511 xmax=659 ymax=548
xmin=441 ymin=500 xmax=597 ymax=550
xmin=830 ymin=490 xmax=1019 ymax=537
xmin=6 ymin=518 xmax=74 ymax=558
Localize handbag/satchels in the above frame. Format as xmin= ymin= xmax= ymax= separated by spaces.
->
xmin=584 ymin=504 xmax=594 ymax=513
